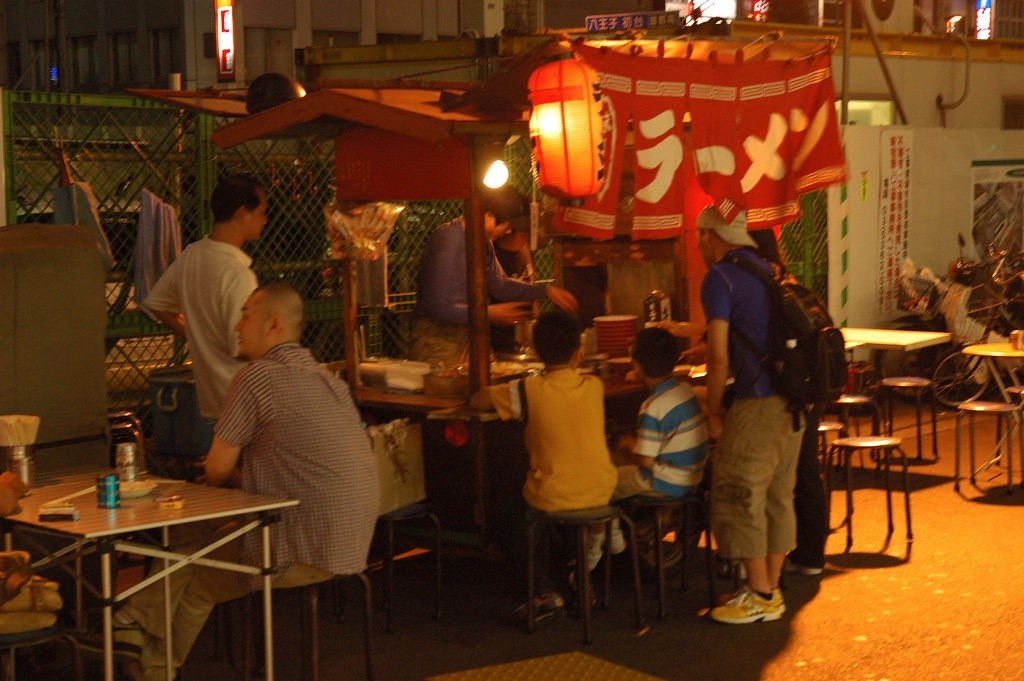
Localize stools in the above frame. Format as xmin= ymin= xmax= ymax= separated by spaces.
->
xmin=524 ymin=504 xmax=644 ymax=644
xmin=816 ymin=421 xmax=853 ymax=534
xmin=632 ymin=494 xmax=720 ymax=619
xmin=956 ymin=403 xmax=1024 ymax=494
xmin=822 ymin=395 xmax=880 ymax=439
xmin=0 ymin=611 xmax=84 ymax=681
xmin=378 ymin=509 xmax=444 ymax=635
xmin=827 ymin=437 xmax=913 ymax=546
xmin=880 ymin=376 xmax=943 ymax=463
xmin=267 ymin=552 xmax=384 ymax=681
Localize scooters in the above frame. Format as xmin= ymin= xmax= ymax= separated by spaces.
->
xmin=872 ymin=232 xmax=1023 ymax=406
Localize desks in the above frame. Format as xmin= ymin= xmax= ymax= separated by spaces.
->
xmin=961 ymin=342 xmax=1024 ymax=486
xmin=834 ymin=326 xmax=952 ymax=459
xmin=0 ymin=467 xmax=299 ymax=681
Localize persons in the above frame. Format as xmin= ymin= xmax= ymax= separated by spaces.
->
xmin=76 ymin=281 xmax=382 ymax=681
xmin=0 ymin=471 xmax=29 ymax=516
xmin=698 ymin=202 xmax=830 ymax=624
xmin=140 ymin=174 xmax=268 ymax=433
xmin=469 ymin=312 xmax=619 ymax=623
xmin=580 ymin=327 xmax=710 ymax=574
xmin=410 ymin=183 xmax=578 ymax=532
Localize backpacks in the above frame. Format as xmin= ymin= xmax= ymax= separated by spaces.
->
xmin=719 ymin=256 xmax=849 ymax=408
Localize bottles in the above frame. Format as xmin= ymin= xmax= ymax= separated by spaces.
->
xmin=108 ymin=411 xmax=146 ymax=481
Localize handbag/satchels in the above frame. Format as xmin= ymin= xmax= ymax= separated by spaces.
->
xmin=367 ymin=418 xmax=426 ymax=516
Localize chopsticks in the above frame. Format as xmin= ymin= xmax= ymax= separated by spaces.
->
xmin=143 ymin=480 xmax=185 ymax=483
xmin=41 ymin=485 xmax=97 ymax=507
xmin=0 ymin=415 xmax=40 ymax=446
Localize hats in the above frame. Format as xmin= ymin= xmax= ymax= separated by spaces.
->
xmin=696 ymin=202 xmax=757 ymax=247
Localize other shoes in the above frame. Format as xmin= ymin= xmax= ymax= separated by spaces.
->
xmin=719 ymin=560 xmax=747 ymax=578
xmin=611 ymin=532 xmax=627 ymax=554
xmin=782 ymin=556 xmax=822 ymax=575
xmin=513 ymin=592 xmax=564 ymax=625
xmin=663 ymin=542 xmax=684 ymax=567
xmin=586 ymin=551 xmax=606 ymax=571
xmin=569 ymin=569 xmax=598 ymax=608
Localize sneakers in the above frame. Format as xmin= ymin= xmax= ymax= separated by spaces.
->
xmin=710 ymin=586 xmax=782 ymax=623
xmin=718 ymin=585 xmax=786 ymax=613
xmin=62 ymin=617 xmax=145 ymax=661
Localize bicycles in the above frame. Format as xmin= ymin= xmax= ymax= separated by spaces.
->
xmin=930 ymin=249 xmax=1024 ymax=410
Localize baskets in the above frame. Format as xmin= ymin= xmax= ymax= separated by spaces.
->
xmin=896 ymin=275 xmax=934 ymax=315
xmin=945 ymin=283 xmax=1005 ymax=345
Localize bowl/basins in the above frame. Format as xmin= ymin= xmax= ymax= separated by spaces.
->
xmin=645 ymin=322 xmax=707 ymax=347
xmin=119 ymin=481 xmax=157 ymax=499
xmin=489 ymin=318 xmax=536 ymax=353
xmin=593 ymin=316 xmax=638 ymax=357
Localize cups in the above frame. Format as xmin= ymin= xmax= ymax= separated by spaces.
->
xmin=7 ymin=446 xmax=34 ymax=488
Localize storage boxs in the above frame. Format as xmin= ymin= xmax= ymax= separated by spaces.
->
xmin=149 ymin=366 xmax=218 ymax=462
